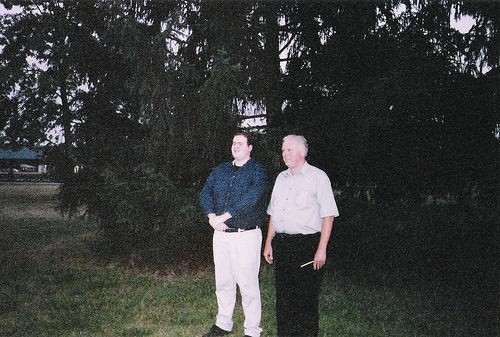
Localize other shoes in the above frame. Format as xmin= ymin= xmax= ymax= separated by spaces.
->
xmin=241 ymin=335 xmax=251 ymax=337
xmin=202 ymin=324 xmax=231 ymax=337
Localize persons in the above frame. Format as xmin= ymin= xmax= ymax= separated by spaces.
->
xmin=263 ymin=134 xmax=339 ymax=337
xmin=199 ymin=131 xmax=272 ymax=337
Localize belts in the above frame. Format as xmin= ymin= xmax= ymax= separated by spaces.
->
xmin=275 ymin=233 xmax=320 ymax=239
xmin=223 ymin=226 xmax=256 ymax=232
